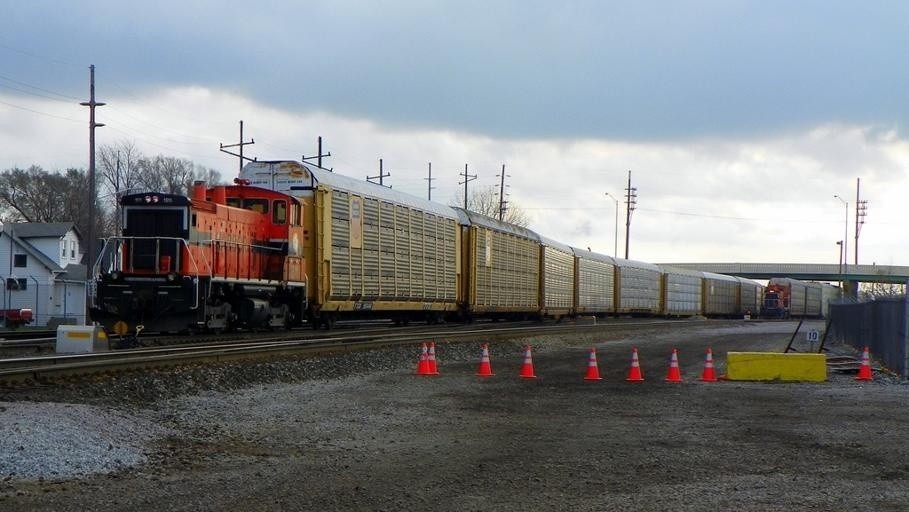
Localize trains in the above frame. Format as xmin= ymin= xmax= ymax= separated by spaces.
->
xmin=759 ymin=277 xmax=877 ymax=324
xmin=86 ymin=158 xmax=764 ymax=342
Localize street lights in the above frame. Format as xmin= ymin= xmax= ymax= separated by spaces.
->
xmin=603 ymin=192 xmax=618 ymax=258
xmin=834 ymin=193 xmax=849 ymax=305
xmin=835 ymin=240 xmax=843 ymax=305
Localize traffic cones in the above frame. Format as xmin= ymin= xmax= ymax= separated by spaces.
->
xmin=854 ymin=345 xmax=875 ymax=383
